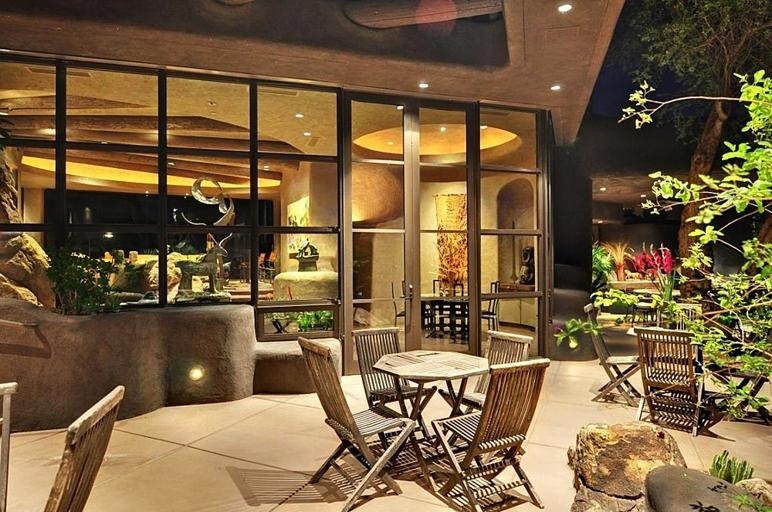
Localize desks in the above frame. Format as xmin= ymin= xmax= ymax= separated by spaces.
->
xmin=370 ymin=347 xmax=491 ymax=460
xmin=421 ymin=295 xmax=470 ymax=346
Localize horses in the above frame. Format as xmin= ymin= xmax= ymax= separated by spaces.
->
xmin=174 ymin=243 xmax=229 ymax=293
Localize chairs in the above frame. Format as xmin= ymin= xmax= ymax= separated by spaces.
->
xmin=418 ymin=358 xmax=554 ymax=512
xmin=657 ymin=302 xmax=704 ymax=330
xmin=41 ymin=382 xmax=127 ymax=512
xmin=352 ymin=326 xmax=434 ymax=450
xmin=296 ymin=335 xmax=416 ymax=511
xmin=632 ymin=326 xmax=708 ymax=436
xmin=390 ymin=280 xmax=404 ymax=326
xmin=420 ymin=330 xmax=533 ymax=449
xmin=481 ymin=280 xmax=501 ymax=330
xmin=583 ymin=300 xmax=640 ymax=403
